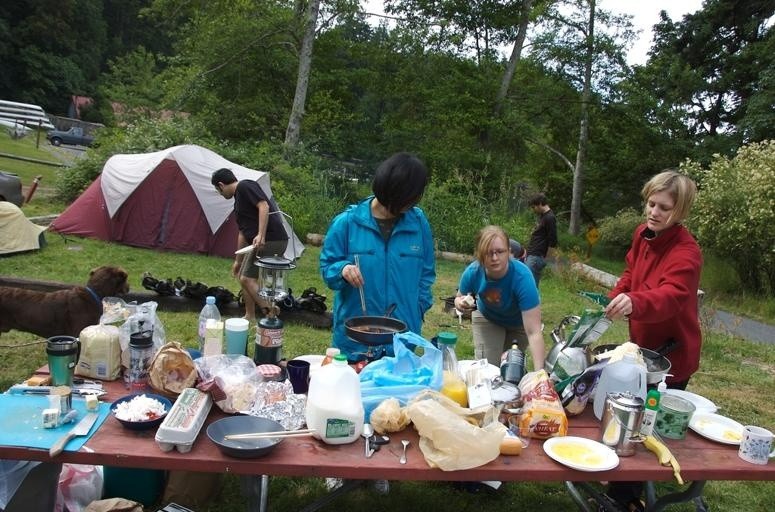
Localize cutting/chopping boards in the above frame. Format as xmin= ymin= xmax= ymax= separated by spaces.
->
xmin=0 ymin=394 xmax=113 ymax=452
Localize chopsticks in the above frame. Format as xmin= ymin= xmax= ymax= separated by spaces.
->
xmin=224 ymin=428 xmax=318 ymax=440
xmin=354 ymin=255 xmax=366 ymax=311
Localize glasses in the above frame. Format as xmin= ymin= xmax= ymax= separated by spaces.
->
xmin=487 ymin=250 xmax=509 ymax=257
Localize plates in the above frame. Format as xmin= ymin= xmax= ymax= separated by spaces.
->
xmin=234 ymin=244 xmax=257 ymax=255
xmin=667 ymin=388 xmax=717 ymax=413
xmin=542 ymin=436 xmax=620 ymax=472
xmin=688 ymin=412 xmax=744 ymax=445
xmin=458 ymin=359 xmax=501 ymax=383
xmin=293 ymin=354 xmax=325 ymax=377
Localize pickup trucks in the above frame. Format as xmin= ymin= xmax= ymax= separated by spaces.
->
xmin=47 ymin=126 xmax=96 ymax=148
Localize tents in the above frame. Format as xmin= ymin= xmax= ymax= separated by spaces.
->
xmin=46 ymin=143 xmax=304 ymax=262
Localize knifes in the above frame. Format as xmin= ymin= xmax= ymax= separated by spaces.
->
xmin=49 ymin=412 xmax=99 ymax=458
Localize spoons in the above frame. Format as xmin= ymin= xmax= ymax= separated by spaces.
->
xmin=360 ymin=423 xmax=374 ymax=457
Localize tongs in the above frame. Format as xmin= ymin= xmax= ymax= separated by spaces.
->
xmin=10 ymin=387 xmax=108 ymax=398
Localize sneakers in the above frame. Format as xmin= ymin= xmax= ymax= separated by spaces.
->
xmin=242 ymin=316 xmax=257 ymax=325
xmin=270 ymin=304 xmax=281 ymax=315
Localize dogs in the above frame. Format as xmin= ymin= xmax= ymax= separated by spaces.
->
xmin=0 ymin=266 xmax=130 ymax=339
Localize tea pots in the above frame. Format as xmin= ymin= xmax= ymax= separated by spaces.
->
xmin=544 ymin=314 xmax=592 ymax=382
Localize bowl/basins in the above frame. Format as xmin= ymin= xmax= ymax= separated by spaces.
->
xmin=205 ymin=416 xmax=287 ymax=459
xmin=109 ymin=392 xmax=172 ymax=429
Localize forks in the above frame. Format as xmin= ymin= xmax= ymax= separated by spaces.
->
xmin=400 ymin=439 xmax=410 ymax=464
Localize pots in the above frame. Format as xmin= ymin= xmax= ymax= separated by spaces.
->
xmin=592 ymin=338 xmax=677 ymax=385
xmin=344 ymin=303 xmax=408 ymax=344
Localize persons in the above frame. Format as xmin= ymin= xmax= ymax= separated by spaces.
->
xmin=319 ymin=151 xmax=437 ymax=362
xmin=211 ymin=169 xmax=289 ymax=324
xmin=524 ymin=196 xmax=558 ymax=287
xmin=452 ymin=225 xmax=545 ymax=377
xmin=507 ymin=238 xmax=525 ymax=263
xmin=595 ymin=169 xmax=704 ymax=507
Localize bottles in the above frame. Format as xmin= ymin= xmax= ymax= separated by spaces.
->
xmin=128 ymin=329 xmax=154 ymax=395
xmin=639 ymin=389 xmax=661 ymax=436
xmin=500 ymin=339 xmax=527 ymax=386
xmin=321 ymin=348 xmax=341 ymax=368
xmin=199 ymin=296 xmax=222 ymax=354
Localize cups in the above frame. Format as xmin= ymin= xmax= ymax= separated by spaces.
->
xmin=431 ymin=337 xmax=451 ymax=361
xmin=508 ymin=415 xmax=538 ymax=449
xmin=284 ymin=360 xmax=310 ymax=394
xmin=46 ymin=335 xmax=82 ymax=386
xmin=225 ymin=318 xmax=250 ymax=359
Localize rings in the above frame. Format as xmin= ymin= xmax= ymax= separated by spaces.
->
xmin=622 ymin=308 xmax=626 ymax=315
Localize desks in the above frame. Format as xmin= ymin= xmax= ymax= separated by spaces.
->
xmin=0 ymin=362 xmax=775 ymax=512
